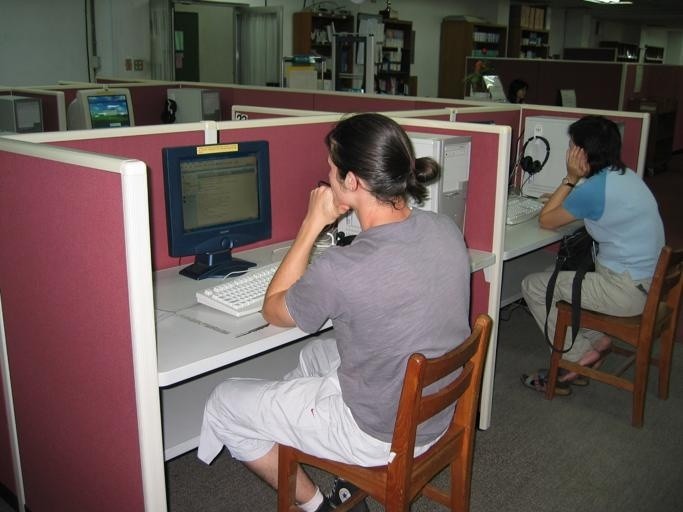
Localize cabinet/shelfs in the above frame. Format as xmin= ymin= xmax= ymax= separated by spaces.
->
xmin=510 ymin=0 xmax=551 ymax=58
xmin=292 ymin=7 xmax=352 ymax=88
xmin=439 ymin=16 xmax=506 ymax=101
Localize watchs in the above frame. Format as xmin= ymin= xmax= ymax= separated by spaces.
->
xmin=562 ymin=178 xmax=575 ymax=188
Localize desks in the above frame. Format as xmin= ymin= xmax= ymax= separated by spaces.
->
xmin=503 ymin=196 xmax=586 ymax=261
xmin=151 ymin=236 xmax=496 ymax=390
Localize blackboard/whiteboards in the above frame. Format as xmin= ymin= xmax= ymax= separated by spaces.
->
xmin=286 ymin=67 xmax=318 ymax=90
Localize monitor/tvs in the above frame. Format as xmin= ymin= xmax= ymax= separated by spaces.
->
xmin=66 ymin=87 xmax=136 ymax=129
xmin=161 ymin=139 xmax=273 ymax=281
xmin=553 ymin=87 xmax=579 ymax=109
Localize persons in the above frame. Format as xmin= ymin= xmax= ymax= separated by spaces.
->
xmin=197 ymin=114 xmax=472 ymax=512
xmin=374 ymin=74 xmax=387 ymax=94
xmin=508 ymin=79 xmax=531 ymax=104
xmin=522 ymin=115 xmax=667 ymax=383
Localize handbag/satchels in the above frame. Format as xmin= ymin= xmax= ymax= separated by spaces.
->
xmin=542 ymin=224 xmax=598 ymax=354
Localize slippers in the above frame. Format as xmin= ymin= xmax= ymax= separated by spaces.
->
xmin=520 ymin=371 xmax=570 ymax=395
xmin=569 ymin=376 xmax=589 ymax=386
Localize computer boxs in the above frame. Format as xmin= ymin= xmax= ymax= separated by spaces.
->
xmin=335 ymin=129 xmax=473 ymax=252
xmin=520 ymin=114 xmax=626 ymax=199
xmin=166 ymin=86 xmax=222 ymax=124
xmin=0 ymin=93 xmax=45 ymax=133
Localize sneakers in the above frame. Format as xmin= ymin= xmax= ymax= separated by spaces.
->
xmin=313 ymin=494 xmax=334 ymax=512
xmin=327 ymin=478 xmax=368 ymax=512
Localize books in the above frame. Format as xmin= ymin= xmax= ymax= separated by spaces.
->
xmin=390 ymin=76 xmax=409 ymax=95
xmin=521 ymin=6 xmax=547 ymax=30
xmin=473 ymin=31 xmax=500 ymax=42
xmin=357 ymin=42 xmax=406 ymax=71
xmin=359 ymin=19 xmax=405 ymax=47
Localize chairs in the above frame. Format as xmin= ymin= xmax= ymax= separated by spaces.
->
xmin=278 ymin=314 xmax=494 ymax=511
xmin=546 ymin=241 xmax=683 ymax=429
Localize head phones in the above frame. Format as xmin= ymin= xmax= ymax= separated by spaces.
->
xmin=159 ymin=97 xmax=178 ymax=125
xmin=335 ymin=231 xmax=356 ymax=246
xmin=516 ymin=135 xmax=551 ymax=177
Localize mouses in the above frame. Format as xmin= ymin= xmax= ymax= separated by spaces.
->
xmin=539 ymin=188 xmax=555 ymax=201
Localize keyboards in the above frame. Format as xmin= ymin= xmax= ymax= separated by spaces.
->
xmin=194 ymin=259 xmax=287 ymax=320
xmin=505 ymin=192 xmax=547 ymax=225
